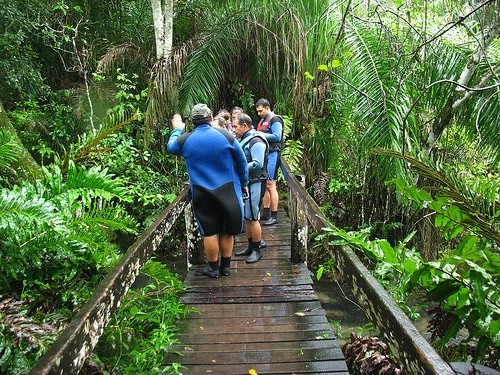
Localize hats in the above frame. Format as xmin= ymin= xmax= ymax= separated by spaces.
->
xmin=190 ymin=103 xmax=211 ymax=119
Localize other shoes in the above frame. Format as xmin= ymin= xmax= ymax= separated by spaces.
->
xmin=202 ymin=266 xmax=231 ymax=278
xmin=258 ymin=241 xmax=266 ymax=248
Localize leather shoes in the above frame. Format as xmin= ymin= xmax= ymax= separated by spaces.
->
xmin=264 ymin=217 xmax=278 ymax=225
xmin=246 ymin=251 xmax=262 ymax=262
xmin=235 ymin=247 xmax=253 ymax=256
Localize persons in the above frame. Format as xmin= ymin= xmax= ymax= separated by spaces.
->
xmin=232 ymin=113 xmax=270 ymax=263
xmin=167 ymin=103 xmax=250 ymax=278
xmin=211 ymin=106 xmax=243 ymax=139
xmin=255 ymin=98 xmax=284 ymax=225
